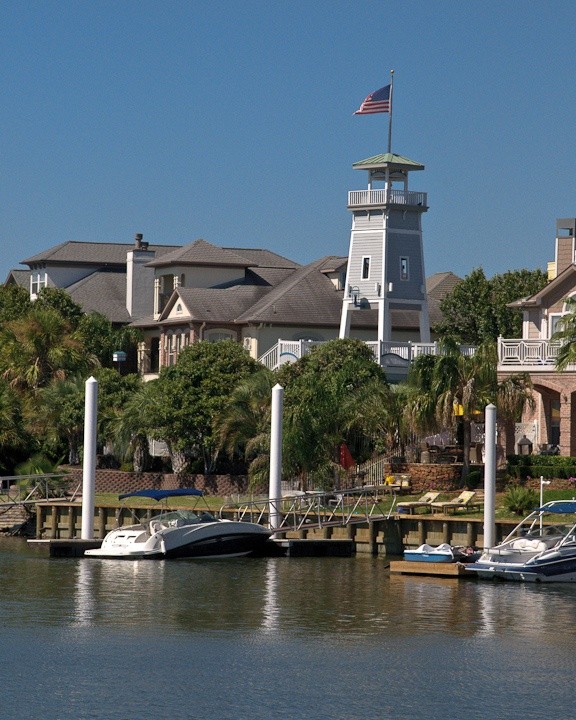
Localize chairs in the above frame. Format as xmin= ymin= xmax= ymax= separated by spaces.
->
xmin=150 ymin=520 xmax=167 ymax=535
xmin=431 ymin=491 xmax=480 ymax=514
xmin=397 ymin=492 xmax=440 ymax=515
xmin=328 ymin=493 xmax=344 ymax=507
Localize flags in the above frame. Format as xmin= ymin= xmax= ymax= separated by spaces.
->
xmin=353 ymin=83 xmax=392 ymax=115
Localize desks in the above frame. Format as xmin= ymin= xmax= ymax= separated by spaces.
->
xmin=306 ymin=490 xmax=325 ymax=509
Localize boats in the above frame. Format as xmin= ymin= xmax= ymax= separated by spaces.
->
xmin=402 ymin=542 xmax=482 ymax=563
xmin=464 ymin=475 xmax=576 ymax=585
xmin=83 ymin=490 xmax=275 ymax=561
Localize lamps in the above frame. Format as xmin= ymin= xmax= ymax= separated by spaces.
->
xmin=420 ymin=285 xmax=426 ymax=293
xmin=518 ymin=435 xmax=532 ymax=458
xmin=389 ymin=282 xmax=393 ymax=292
xmin=347 ymin=284 xmax=368 ymax=309
xmin=375 ymin=282 xmax=379 ymax=291
